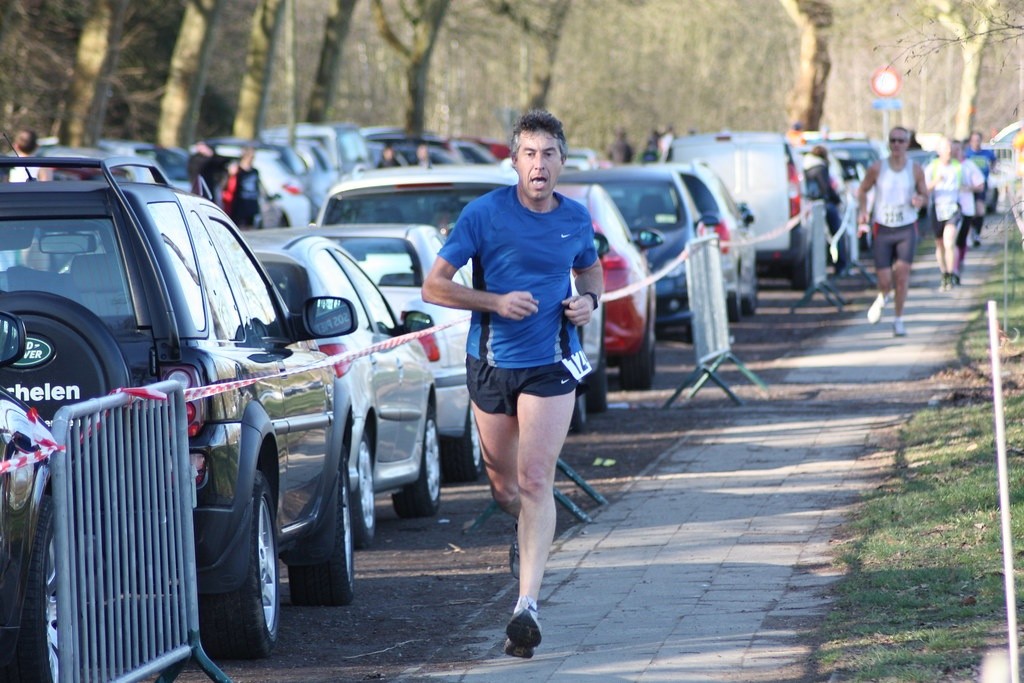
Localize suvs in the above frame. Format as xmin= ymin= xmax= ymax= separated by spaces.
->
xmin=0 ymin=152 xmax=358 ymax=654
xmin=315 ymin=157 xmax=518 ymax=280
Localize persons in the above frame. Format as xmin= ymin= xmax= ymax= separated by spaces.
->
xmin=9 ymin=130 xmax=48 ymax=183
xmin=416 ymin=144 xmax=432 ymax=164
xmin=189 ymin=141 xmax=259 ymax=228
xmin=377 ymin=146 xmax=401 ymax=169
xmin=924 ymin=130 xmax=997 ymax=291
xmin=644 ymin=130 xmax=669 ymax=161
xmin=858 ymin=127 xmax=929 ymax=336
xmin=419 ymin=110 xmax=605 ymax=661
xmin=608 ymin=127 xmax=631 ymax=163
xmin=786 ymin=122 xmax=806 ymax=147
xmin=804 ymin=147 xmax=847 ymax=275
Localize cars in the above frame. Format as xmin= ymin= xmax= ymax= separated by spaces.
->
xmin=555 ymin=172 xmax=755 ymax=343
xmin=551 ymin=186 xmax=659 ymax=391
xmin=802 ymin=144 xmax=932 ymax=247
xmin=105 ymin=129 xmax=499 ymax=227
xmin=239 ymin=231 xmax=443 ymax=548
xmin=311 ymin=224 xmax=482 ymax=477
xmin=0 ymin=313 xmax=58 ymax=683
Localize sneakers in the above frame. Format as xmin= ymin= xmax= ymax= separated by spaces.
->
xmin=510 ymin=519 xmax=521 ymax=579
xmin=895 ymin=322 xmax=906 ymax=334
xmin=504 ymin=609 xmax=542 ymax=658
xmin=867 ymin=296 xmax=889 ymax=324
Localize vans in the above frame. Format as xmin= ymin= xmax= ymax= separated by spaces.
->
xmin=665 ymin=130 xmax=815 ymax=287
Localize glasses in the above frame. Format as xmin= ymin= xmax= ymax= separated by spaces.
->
xmin=890 ymin=137 xmax=906 ymax=143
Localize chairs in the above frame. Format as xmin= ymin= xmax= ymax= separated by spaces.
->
xmin=637 ymin=193 xmax=668 ymax=217
xmin=71 ymin=253 xmax=121 ymax=296
xmin=372 ymin=206 xmax=406 ymax=224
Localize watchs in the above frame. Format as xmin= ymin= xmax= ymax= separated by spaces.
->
xmin=583 ymin=292 xmax=598 ymax=310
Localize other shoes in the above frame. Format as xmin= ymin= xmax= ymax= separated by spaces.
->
xmin=940 ymin=278 xmax=952 ymax=290
